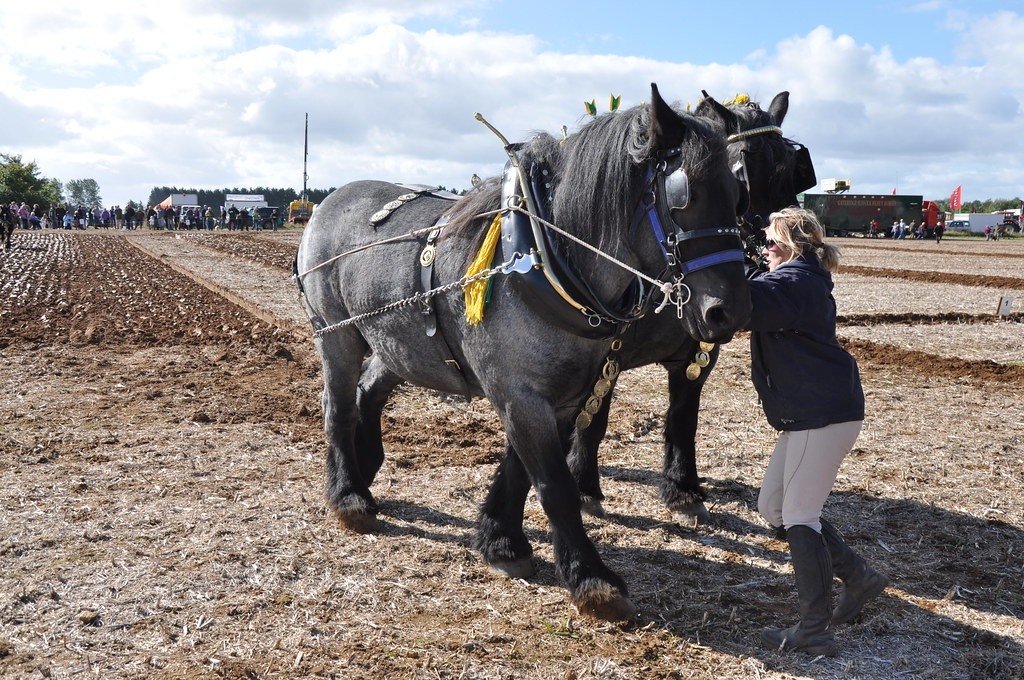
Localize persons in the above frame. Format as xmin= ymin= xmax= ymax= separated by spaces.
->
xmin=76 ymin=206 xmax=87 ymax=230
xmin=193 ymin=207 xmax=201 ymax=231
xmin=101 ymin=207 xmax=110 ymax=230
xmin=863 ymin=219 xmax=879 ymax=239
xmin=984 ymin=224 xmax=992 ymax=241
xmin=186 ymin=207 xmax=194 ymax=229
xmin=891 ymin=219 xmax=906 ymax=240
xmin=49 ymin=203 xmax=74 ymax=230
xmin=201 ymin=204 xmax=214 ymax=231
xmin=252 ymin=206 xmax=260 ymax=231
xmin=909 ymin=220 xmax=916 ymax=240
xmin=124 ymin=205 xmax=144 ymax=230
xmin=88 ymin=205 xmax=100 ymax=230
xmin=146 ymin=204 xmax=181 ymax=230
xmin=10 ymin=201 xmax=50 ymax=231
xmin=228 ymin=204 xmax=239 ymax=231
xmin=220 ymin=207 xmax=228 ymax=229
xmin=994 ymin=222 xmax=999 ymax=241
xmin=240 ymin=207 xmax=250 ymax=231
xmin=1003 ymin=227 xmax=1010 ymax=239
xmin=933 ymin=222 xmax=944 ymax=244
xmin=916 ymin=222 xmax=926 ymax=240
xmin=269 ymin=208 xmax=279 ymax=231
xmin=110 ymin=205 xmax=123 ymax=230
xmin=747 ymin=204 xmax=892 ymax=657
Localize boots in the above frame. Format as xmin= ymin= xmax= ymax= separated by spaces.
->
xmin=775 ymin=521 xmax=888 ymax=625
xmin=760 ymin=524 xmax=837 ymax=658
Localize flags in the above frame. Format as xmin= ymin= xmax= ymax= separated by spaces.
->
xmin=950 ymin=184 xmax=962 ymax=211
xmin=891 ymin=187 xmax=897 ymax=195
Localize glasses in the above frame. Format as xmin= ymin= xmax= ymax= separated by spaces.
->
xmin=762 ymin=238 xmax=783 ymax=249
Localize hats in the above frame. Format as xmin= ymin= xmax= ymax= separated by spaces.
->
xmin=937 ymin=222 xmax=941 ymax=225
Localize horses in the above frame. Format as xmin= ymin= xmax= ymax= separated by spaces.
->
xmin=298 ymin=81 xmax=792 ymax=621
